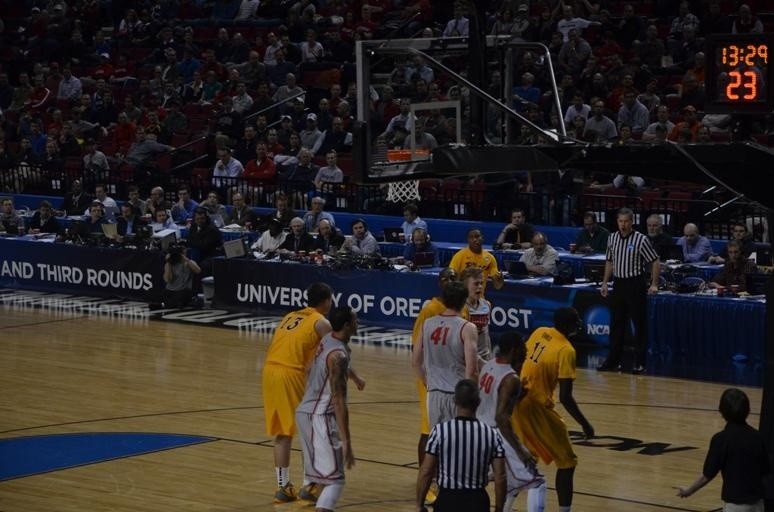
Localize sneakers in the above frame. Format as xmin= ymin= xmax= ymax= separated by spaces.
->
xmin=424 ymin=480 xmax=440 ymax=504
xmin=273 ymin=480 xmax=323 ymax=505
xmin=632 ymin=363 xmax=647 ymax=375
xmin=597 ymin=360 xmax=622 ymax=370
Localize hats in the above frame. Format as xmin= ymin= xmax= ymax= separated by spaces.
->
xmin=306 ymin=112 xmax=317 ymax=121
xmin=293 ymin=97 xmax=304 ymax=103
xmin=280 ymin=114 xmax=292 ymax=122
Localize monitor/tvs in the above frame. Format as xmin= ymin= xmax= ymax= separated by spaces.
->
xmin=583 ymin=265 xmax=613 ymax=286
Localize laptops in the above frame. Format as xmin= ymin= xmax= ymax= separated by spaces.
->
xmin=655 ymin=243 xmax=690 ymax=264
xmin=503 ymin=259 xmax=531 ymax=277
xmin=384 ymin=227 xmax=406 ymax=243
xmin=555 ymin=259 xmax=589 ymax=283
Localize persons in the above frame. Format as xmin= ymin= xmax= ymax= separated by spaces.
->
xmin=475 ymin=333 xmax=547 ymax=512
xmin=262 ymin=283 xmax=366 ymax=505
xmin=410 ymin=270 xmax=471 ymax=504
xmin=1 ymin=2 xmax=773 ymax=377
xmin=509 ymin=307 xmax=595 ymax=512
xmin=416 ymin=380 xmax=508 ymax=512
xmin=412 ymin=281 xmax=478 ymax=493
xmin=295 ymin=305 xmax=356 ymax=512
xmin=671 ymin=388 xmax=770 ymax=512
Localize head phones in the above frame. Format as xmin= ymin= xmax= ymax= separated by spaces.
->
xmin=409 ymin=227 xmax=431 ymax=243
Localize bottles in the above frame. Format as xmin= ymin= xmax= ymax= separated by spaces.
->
xmin=716 ymin=283 xmax=740 ymax=297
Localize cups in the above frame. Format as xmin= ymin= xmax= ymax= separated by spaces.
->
xmin=381 ymin=255 xmax=409 ymax=271
xmin=185 ymin=219 xmax=190 ymax=229
xmin=279 ymin=248 xmax=329 ymax=265
xmin=569 ymin=242 xmax=594 ymax=256
xmin=243 ymin=222 xmax=253 ymax=231
xmin=16 ymin=225 xmax=39 ymax=240
xmin=145 ymin=214 xmax=152 ymax=226
